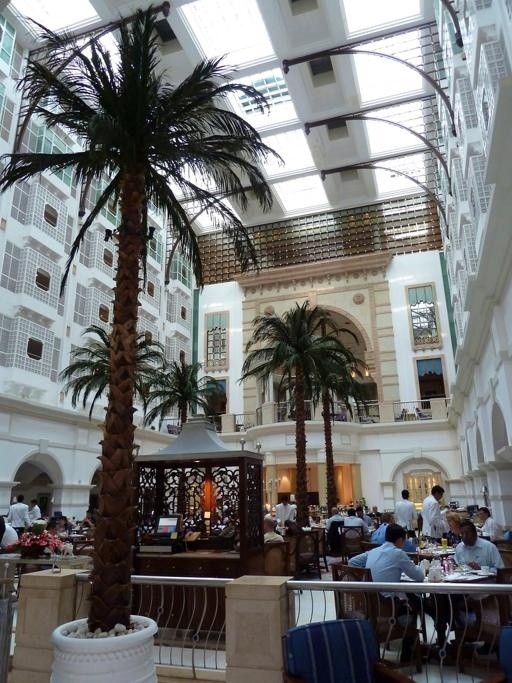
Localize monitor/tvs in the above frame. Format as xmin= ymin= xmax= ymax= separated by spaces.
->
xmin=153 ymin=515 xmax=181 ymax=536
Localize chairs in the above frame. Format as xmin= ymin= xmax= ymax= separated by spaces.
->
xmin=266 ymin=506 xmax=512 ymax=672
xmin=0 ymin=511 xmax=98 ymax=602
xmin=282 ymin=620 xmax=413 ymax=683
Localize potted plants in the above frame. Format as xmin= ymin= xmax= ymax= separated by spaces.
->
xmin=0 ymin=3 xmax=274 ymax=681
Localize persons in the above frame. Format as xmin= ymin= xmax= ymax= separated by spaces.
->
xmin=0 ymin=494 xmax=238 ymax=575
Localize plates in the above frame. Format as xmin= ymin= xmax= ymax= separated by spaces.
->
xmin=478 ymin=572 xmax=495 ymax=576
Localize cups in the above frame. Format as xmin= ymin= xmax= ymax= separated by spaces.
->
xmin=442 ymin=538 xmax=448 ymax=547
xmin=419 ymin=541 xmax=426 ymax=549
xmin=481 ymin=566 xmax=490 ymax=573
xmin=418 ymin=555 xmax=480 ymax=582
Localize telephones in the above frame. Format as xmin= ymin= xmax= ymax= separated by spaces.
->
xmin=182 ymin=531 xmax=201 ymax=541
